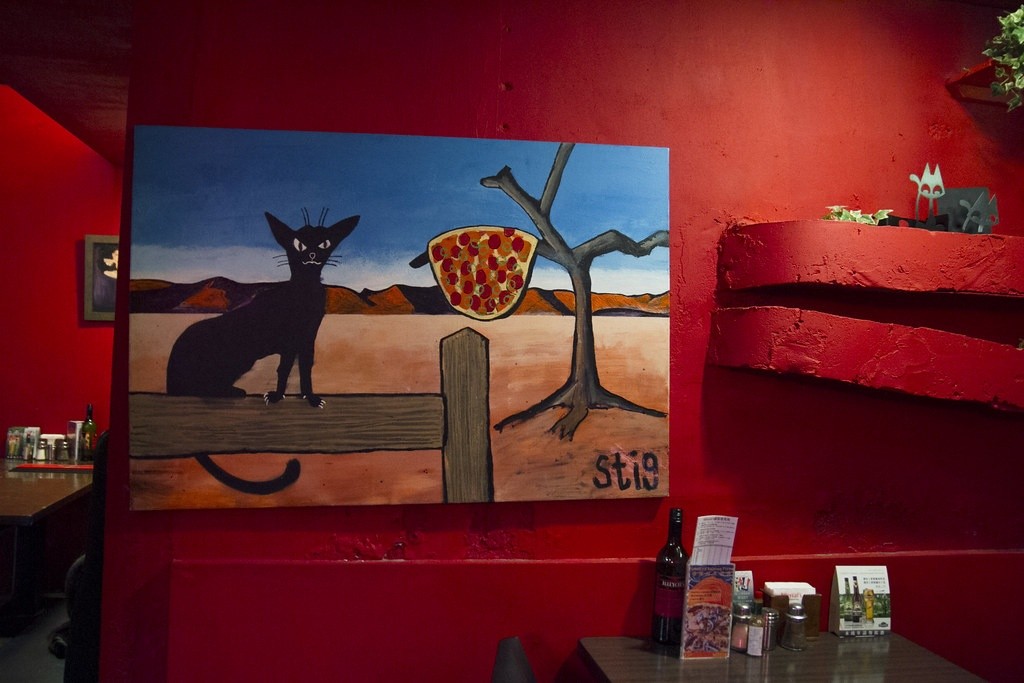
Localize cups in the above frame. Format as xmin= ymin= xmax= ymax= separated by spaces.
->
xmin=862 ymin=589 xmax=874 ymax=624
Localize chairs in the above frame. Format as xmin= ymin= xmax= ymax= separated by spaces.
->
xmin=49 ymin=429 xmax=111 ymax=657
xmin=494 ymin=637 xmax=537 ymax=683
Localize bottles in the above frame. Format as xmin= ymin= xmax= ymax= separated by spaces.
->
xmin=652 ymin=507 xmax=688 ymax=645
xmin=746 ymin=604 xmax=764 ymax=657
xmin=778 ymin=602 xmax=808 ymax=653
xmin=34 ymin=438 xmax=49 ymax=460
xmin=844 ymin=578 xmax=852 ymax=621
xmin=82 ymin=403 xmax=97 ymax=461
xmin=760 ymin=608 xmax=778 ymax=650
xmin=731 ymin=602 xmax=754 ymax=653
xmin=21 ymin=432 xmax=33 ymax=461
xmin=853 ymin=576 xmax=862 ymax=622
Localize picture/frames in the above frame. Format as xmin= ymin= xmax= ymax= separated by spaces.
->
xmin=84 ymin=235 xmax=120 ymax=321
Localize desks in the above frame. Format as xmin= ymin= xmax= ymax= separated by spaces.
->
xmin=0 ymin=458 xmax=100 ymax=636
xmin=578 ymin=629 xmax=986 ymax=683
